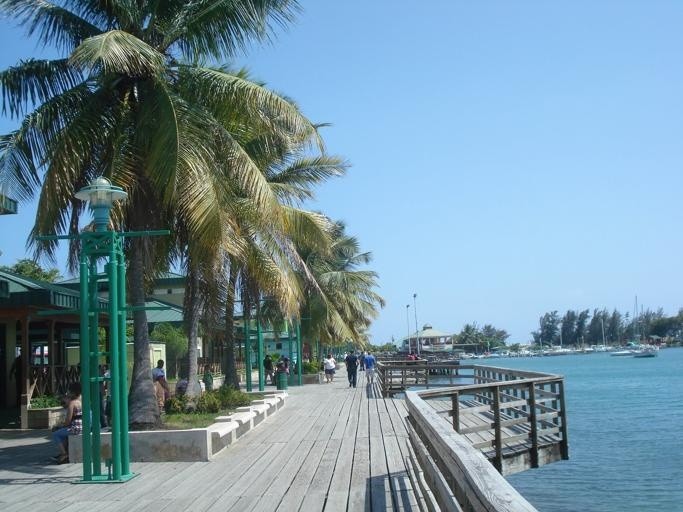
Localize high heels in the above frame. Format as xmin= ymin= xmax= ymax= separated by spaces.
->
xmin=48 ymin=454 xmax=68 ymax=464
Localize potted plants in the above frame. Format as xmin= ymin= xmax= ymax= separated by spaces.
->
xmin=27 ymin=392 xmax=66 ymax=430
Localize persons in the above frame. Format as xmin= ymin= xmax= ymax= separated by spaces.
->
xmin=203 ymin=364 xmax=213 ymax=391
xmin=151 ymin=360 xmax=187 ymax=408
xmin=48 ymin=382 xmax=82 ymax=465
xmin=323 ymin=352 xmax=337 ymax=383
xmin=264 ymin=355 xmax=289 ymax=384
xmin=345 ymin=350 xmax=376 ymax=388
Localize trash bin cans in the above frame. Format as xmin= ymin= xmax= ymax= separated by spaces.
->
xmin=276 ymin=371 xmax=288 ymax=390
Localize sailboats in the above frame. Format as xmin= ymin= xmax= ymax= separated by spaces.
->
xmin=456 ymin=295 xmax=663 ymax=360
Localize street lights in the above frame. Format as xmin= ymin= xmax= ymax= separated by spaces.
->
xmin=405 ymin=303 xmax=413 ymax=356
xmin=67 ymin=173 xmax=144 ymax=487
xmin=412 ymin=292 xmax=422 ymax=357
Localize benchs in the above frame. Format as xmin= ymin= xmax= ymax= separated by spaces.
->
xmin=207 ymin=393 xmax=287 ymax=439
